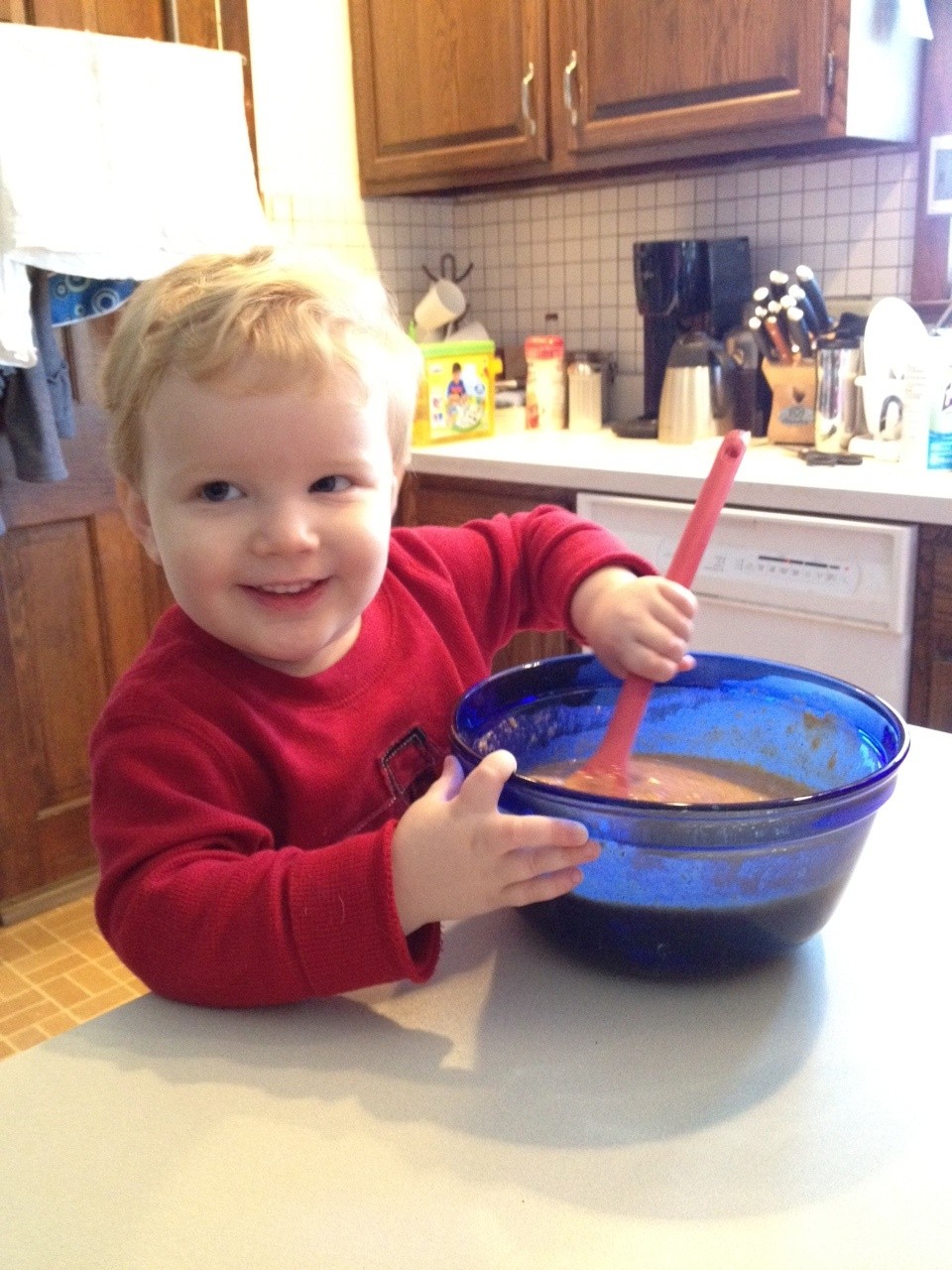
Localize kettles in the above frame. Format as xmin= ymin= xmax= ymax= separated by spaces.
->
xmin=660 ymin=325 xmax=739 ymax=444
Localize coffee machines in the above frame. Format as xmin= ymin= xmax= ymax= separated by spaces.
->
xmin=613 ymin=234 xmax=755 ymax=441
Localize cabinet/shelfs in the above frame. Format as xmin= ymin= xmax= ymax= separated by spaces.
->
xmin=0 ymin=504 xmax=168 ymax=919
xmin=0 ymin=0 xmax=263 ymax=207
xmin=346 ymin=1 xmax=927 ymax=199
xmin=403 ymin=469 xmax=583 ymax=677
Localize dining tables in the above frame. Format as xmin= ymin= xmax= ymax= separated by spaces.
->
xmin=2 ymin=715 xmax=951 ymax=1269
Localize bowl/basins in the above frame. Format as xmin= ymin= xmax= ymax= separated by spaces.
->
xmin=445 ymin=647 xmax=915 ymax=973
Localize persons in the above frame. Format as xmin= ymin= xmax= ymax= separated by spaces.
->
xmin=446 ymin=360 xmax=469 ymax=400
xmin=89 ymin=246 xmax=701 ymax=1013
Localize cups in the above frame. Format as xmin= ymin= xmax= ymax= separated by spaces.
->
xmin=414 ymin=279 xmax=467 ymax=330
